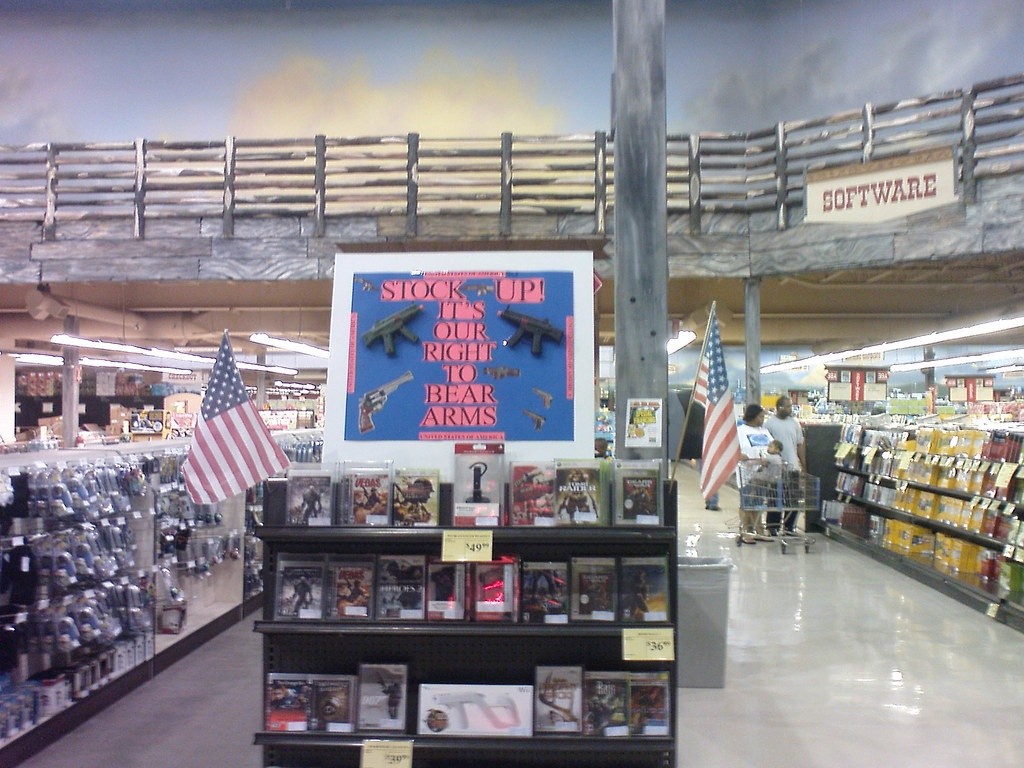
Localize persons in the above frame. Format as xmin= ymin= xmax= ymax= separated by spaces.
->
xmin=762 ymin=396 xmax=806 ymax=537
xmin=735 ymin=405 xmax=779 ymax=545
xmin=704 ymin=493 xmax=721 ymax=510
xmin=748 ymin=440 xmax=783 ymax=496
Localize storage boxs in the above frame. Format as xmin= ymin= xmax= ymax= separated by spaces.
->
xmin=261 ymin=442 xmax=675 ymax=742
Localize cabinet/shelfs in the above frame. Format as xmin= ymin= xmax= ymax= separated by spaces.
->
xmin=252 ymin=479 xmax=678 ymax=768
xmin=826 ymin=427 xmax=1024 ymax=634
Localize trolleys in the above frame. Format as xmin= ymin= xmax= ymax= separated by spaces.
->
xmin=735 ymin=456 xmax=820 ymax=554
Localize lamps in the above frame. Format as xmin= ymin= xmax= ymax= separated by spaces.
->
xmin=11 ymin=272 xmax=332 ymax=375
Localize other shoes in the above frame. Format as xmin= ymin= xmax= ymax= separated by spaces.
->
xmin=740 ymin=535 xmax=756 ymax=544
xmin=755 ymin=536 xmax=774 ymax=542
xmin=784 ymin=527 xmax=798 ymax=536
xmin=767 ymin=527 xmax=777 ymax=536
xmin=705 ymin=505 xmax=721 ymax=510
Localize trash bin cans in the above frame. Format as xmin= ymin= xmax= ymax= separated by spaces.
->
xmin=677 ymin=556 xmax=733 ymax=688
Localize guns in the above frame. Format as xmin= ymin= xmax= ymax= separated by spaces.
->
xmin=361 ymin=302 xmax=425 ymax=356
xmin=500 ymin=304 xmax=565 ymax=356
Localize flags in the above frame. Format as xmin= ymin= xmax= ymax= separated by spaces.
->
xmin=183 ymin=334 xmax=291 ymax=509
xmin=693 ymin=309 xmax=742 ymax=501
